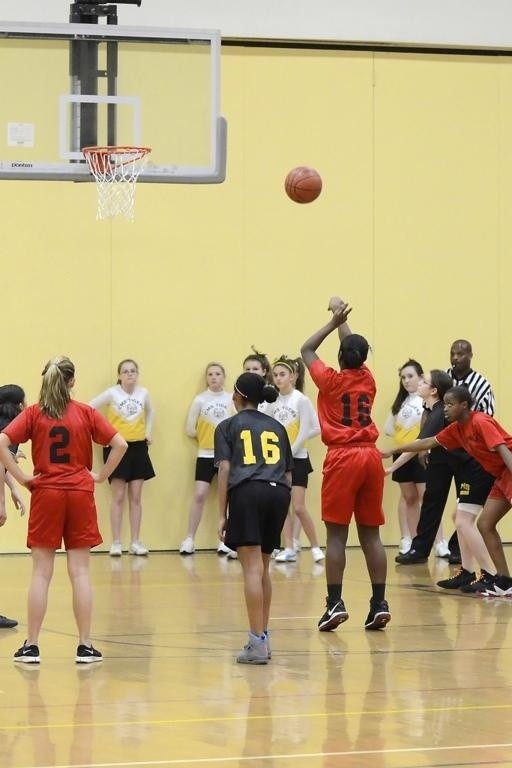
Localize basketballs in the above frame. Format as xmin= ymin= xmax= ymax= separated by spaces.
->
xmin=284 ymin=165 xmax=322 ymax=205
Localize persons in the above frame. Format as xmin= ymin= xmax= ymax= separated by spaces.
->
xmin=264 ymin=355 xmax=325 ymax=562
xmin=0 ymin=385 xmax=26 ymax=632
xmin=214 ymin=372 xmax=293 ymax=665
xmin=395 ymin=340 xmax=495 ymax=565
xmin=0 ymin=360 xmax=129 ymax=665
xmin=384 ymin=369 xmax=497 ymax=594
xmin=286 ymin=357 xmax=321 ymax=553
xmin=300 ymin=296 xmax=392 ymax=631
xmin=227 ymin=355 xmax=272 ymax=561
xmin=85 ymin=360 xmax=155 ymax=556
xmin=180 ymin=363 xmax=239 ymax=556
xmin=386 ymin=360 xmax=452 ymax=557
xmin=380 ymin=384 xmax=512 ymax=596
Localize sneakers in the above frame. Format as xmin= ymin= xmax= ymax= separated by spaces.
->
xmin=434 ymin=540 xmax=450 ymax=559
xmin=225 ymin=550 xmax=238 ymax=560
xmin=217 ymin=541 xmax=229 ymax=553
xmin=75 ymin=644 xmax=103 ymax=665
xmin=11 ymin=640 xmax=42 ymax=665
xmin=476 ymin=575 xmax=512 ymax=599
xmin=1 ymin=614 xmax=19 ymax=630
xmin=265 ymin=631 xmax=271 ymax=660
xmin=317 ymin=597 xmax=349 ymax=631
xmin=448 ymin=552 xmax=462 ymax=565
xmin=235 ymin=632 xmax=269 ymax=665
xmin=292 ymin=539 xmax=301 ymax=552
xmin=109 ymin=543 xmax=122 ymax=558
xmin=277 ymin=550 xmax=298 ymax=563
xmin=397 ymin=535 xmax=413 ymax=555
xmin=362 ymin=597 xmax=392 ymax=631
xmin=311 ymin=546 xmax=326 ymax=563
xmin=434 ymin=566 xmax=476 ymax=597
xmin=179 ymin=537 xmax=195 ymax=556
xmin=392 ymin=548 xmax=427 ymax=564
xmin=128 ymin=540 xmax=151 ymax=558
xmin=458 ymin=568 xmax=494 ymax=597
